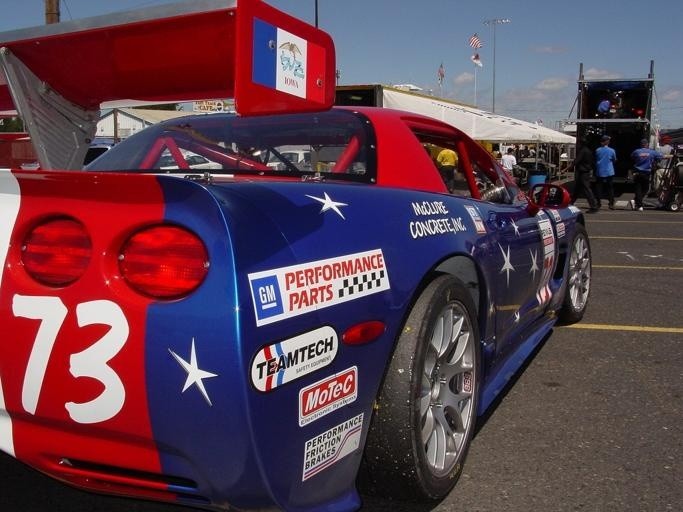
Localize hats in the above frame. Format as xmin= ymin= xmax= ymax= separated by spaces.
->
xmin=640 ymin=139 xmax=648 ymax=144
xmin=600 ymin=135 xmax=611 ymax=142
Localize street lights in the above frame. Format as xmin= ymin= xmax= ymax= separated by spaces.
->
xmin=480 ymin=18 xmax=513 ymax=115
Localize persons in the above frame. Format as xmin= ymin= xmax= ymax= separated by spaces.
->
xmin=561 ymin=136 xmax=599 ymax=214
xmin=595 ymin=135 xmax=617 ymax=209
xmin=630 ymin=139 xmax=682 ymax=213
xmin=437 ymin=146 xmax=459 ymax=194
xmin=500 ymin=148 xmax=517 ymax=173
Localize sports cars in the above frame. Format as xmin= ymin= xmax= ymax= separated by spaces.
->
xmin=0 ymin=1 xmax=592 ymax=510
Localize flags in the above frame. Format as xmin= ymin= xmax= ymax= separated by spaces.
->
xmin=438 ymin=62 xmax=445 ymax=86
xmin=470 ymin=53 xmax=483 ymax=68
xmin=469 ymin=33 xmax=483 ymax=48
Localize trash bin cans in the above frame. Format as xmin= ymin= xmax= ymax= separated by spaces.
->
xmin=530 ymin=175 xmax=545 ymax=189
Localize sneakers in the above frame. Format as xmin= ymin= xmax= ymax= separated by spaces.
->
xmin=636 ymin=206 xmax=644 ymax=211
xmin=629 ymin=199 xmax=635 ymax=210
xmin=586 ymin=208 xmax=598 ymax=213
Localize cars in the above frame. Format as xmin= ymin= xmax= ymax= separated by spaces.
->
xmin=84 ymin=135 xmax=310 ymax=168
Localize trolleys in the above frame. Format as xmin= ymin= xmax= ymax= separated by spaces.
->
xmin=657 ymin=147 xmax=683 ymax=212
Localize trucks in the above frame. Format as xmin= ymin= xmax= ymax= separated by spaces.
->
xmin=574 ymin=60 xmax=655 ymax=199
